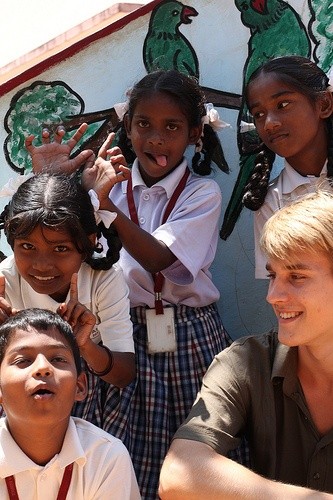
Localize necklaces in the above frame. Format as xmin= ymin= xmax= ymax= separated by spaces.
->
xmin=4 ymin=462 xmax=75 ymax=500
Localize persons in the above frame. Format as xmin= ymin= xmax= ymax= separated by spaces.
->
xmin=0 ymin=170 xmax=137 ymax=387
xmin=24 ymin=68 xmax=234 ymax=500
xmin=242 ymin=55 xmax=332 ymax=279
xmin=0 ymin=307 xmax=142 ymax=500
xmin=157 ymin=190 xmax=333 ymax=500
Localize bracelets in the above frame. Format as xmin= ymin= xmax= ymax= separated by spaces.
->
xmin=86 ymin=345 xmax=114 ymax=376
